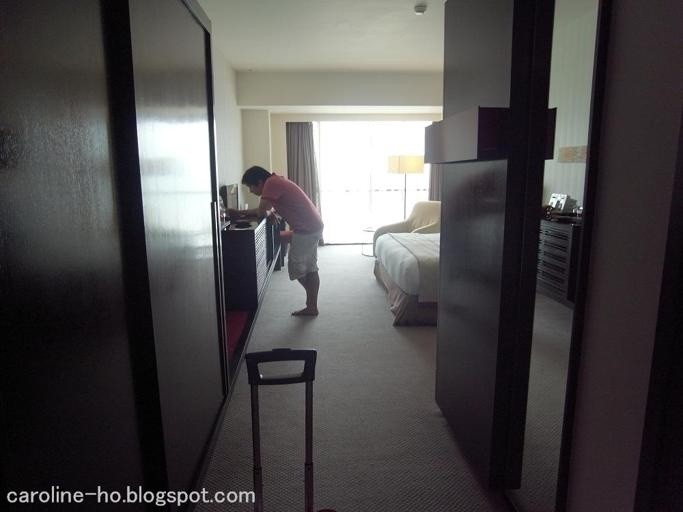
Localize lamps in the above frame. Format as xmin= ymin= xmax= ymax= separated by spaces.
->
xmin=389 ymin=155 xmax=424 ymax=223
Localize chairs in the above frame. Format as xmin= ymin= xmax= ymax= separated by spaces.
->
xmin=373 ymin=200 xmax=441 ymax=258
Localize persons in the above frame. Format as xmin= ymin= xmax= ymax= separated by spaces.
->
xmin=224 ymin=165 xmax=323 ymax=317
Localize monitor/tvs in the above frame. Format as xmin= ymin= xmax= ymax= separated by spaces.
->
xmin=219 ymin=183 xmax=239 ymax=210
xmin=549 ymin=193 xmax=577 ymax=211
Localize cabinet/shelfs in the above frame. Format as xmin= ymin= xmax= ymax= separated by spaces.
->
xmin=0 ymin=0 xmax=279 ymax=512
xmin=534 ymin=218 xmax=583 ymax=311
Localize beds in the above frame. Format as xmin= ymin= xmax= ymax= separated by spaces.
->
xmin=372 ymin=233 xmax=440 ymax=327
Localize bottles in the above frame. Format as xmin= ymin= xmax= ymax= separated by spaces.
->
xmin=218 ymin=195 xmax=227 ymax=219
